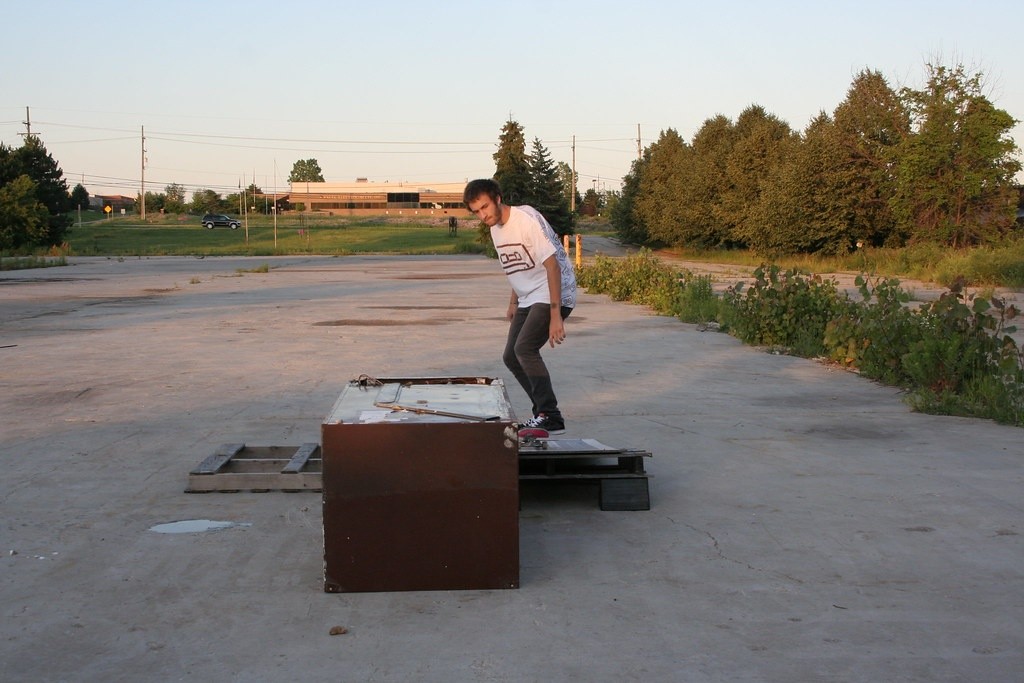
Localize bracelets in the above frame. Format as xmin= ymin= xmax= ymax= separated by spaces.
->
xmin=510 ymin=299 xmax=518 ymax=304
xmin=550 ymin=303 xmax=561 ymax=308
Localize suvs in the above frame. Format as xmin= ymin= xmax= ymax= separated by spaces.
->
xmin=201 ymin=214 xmax=242 ymax=230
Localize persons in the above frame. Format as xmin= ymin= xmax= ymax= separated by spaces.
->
xmin=464 ymin=179 xmax=577 ymax=435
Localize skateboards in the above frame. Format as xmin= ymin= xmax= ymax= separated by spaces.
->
xmin=518 ymin=427 xmax=549 ymax=449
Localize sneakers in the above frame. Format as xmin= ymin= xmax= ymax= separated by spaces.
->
xmin=518 ymin=415 xmax=566 ymax=435
